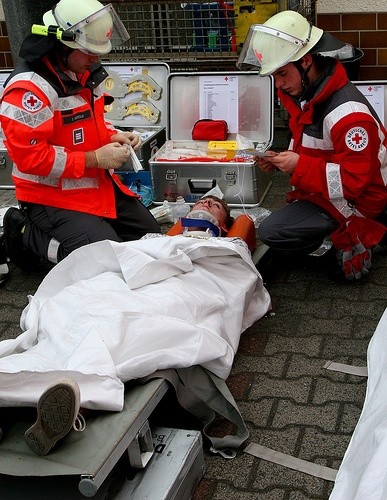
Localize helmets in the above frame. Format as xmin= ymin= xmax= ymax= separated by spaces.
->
xmin=253 ymin=10 xmax=324 ymax=77
xmin=42 ymin=0 xmax=112 ymax=55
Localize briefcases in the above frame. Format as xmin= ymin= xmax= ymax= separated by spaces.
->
xmin=148 ymin=71 xmax=274 ymax=208
xmin=93 ymin=62 xmax=169 ymax=171
xmin=0 ymin=69 xmax=16 ymax=189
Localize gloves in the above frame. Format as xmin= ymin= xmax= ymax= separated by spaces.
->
xmin=330 ymin=213 xmax=371 ymax=280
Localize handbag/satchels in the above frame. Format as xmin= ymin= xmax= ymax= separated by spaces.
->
xmin=192 ymin=119 xmax=229 ymax=141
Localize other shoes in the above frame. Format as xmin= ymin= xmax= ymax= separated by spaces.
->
xmin=335 ymin=244 xmax=382 ymax=283
xmin=24 ymin=379 xmax=86 ymax=456
xmin=3 ymin=207 xmax=37 ymax=273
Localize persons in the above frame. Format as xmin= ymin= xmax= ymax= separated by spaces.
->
xmin=0 ymin=196 xmax=233 ymax=457
xmin=235 ymin=10 xmax=387 ymax=285
xmin=3 ymin=0 xmax=160 ymax=269
